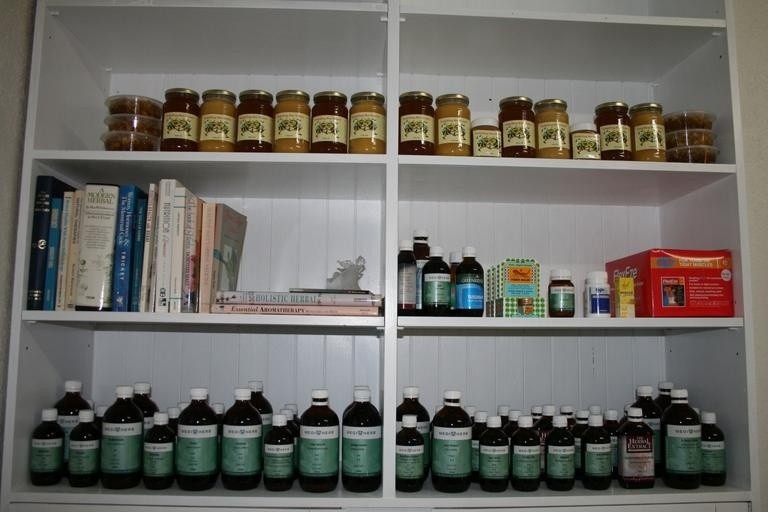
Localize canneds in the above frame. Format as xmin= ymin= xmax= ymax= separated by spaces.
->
xmin=398 ymin=91 xmax=667 ymax=162
xmin=161 ymin=88 xmax=386 ymax=154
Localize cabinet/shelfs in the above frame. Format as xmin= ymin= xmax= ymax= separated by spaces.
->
xmin=0 ymin=0 xmax=761 ymax=512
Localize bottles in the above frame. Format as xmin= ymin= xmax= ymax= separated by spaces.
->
xmin=550 ymin=269 xmax=576 ymax=316
xmin=397 ymin=229 xmax=486 ymax=317
xmin=159 ymin=87 xmax=384 ymax=153
xmin=395 ymin=384 xmax=729 ymax=495
xmin=23 ymin=379 xmax=381 ymax=497
xmin=582 ymin=272 xmax=611 ymax=318
xmin=397 ymin=91 xmax=665 ymax=161
xmin=518 ymin=297 xmax=534 ymax=316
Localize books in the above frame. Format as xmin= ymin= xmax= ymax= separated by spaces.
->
xmin=215 ymin=289 xmax=385 ymax=307
xmin=26 ymin=174 xmax=246 ymax=318
xmin=289 ymin=287 xmax=377 ymax=295
xmin=209 ymin=303 xmax=381 ymax=317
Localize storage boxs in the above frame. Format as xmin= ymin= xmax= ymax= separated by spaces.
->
xmin=605 ymin=249 xmax=735 ymax=318
